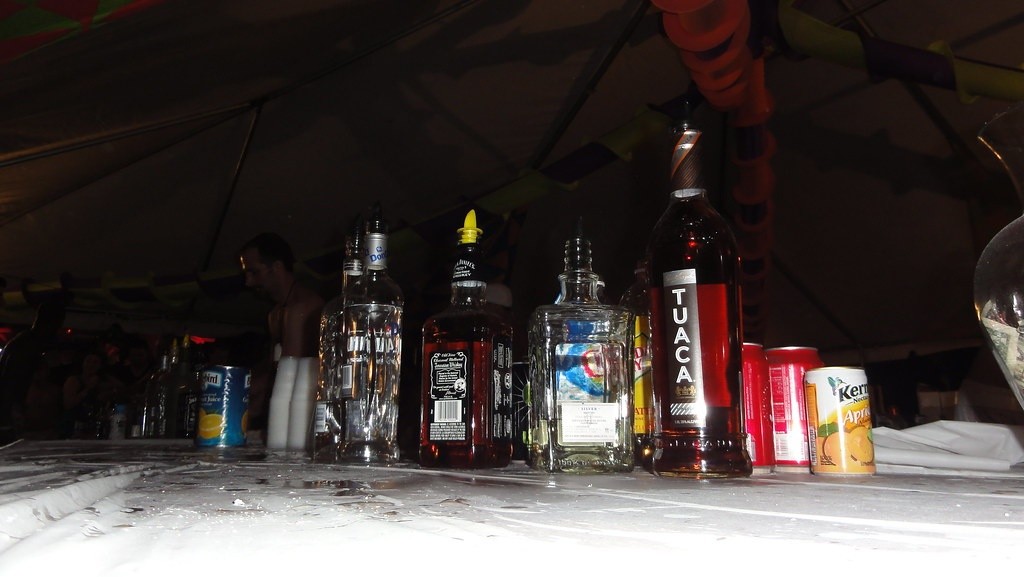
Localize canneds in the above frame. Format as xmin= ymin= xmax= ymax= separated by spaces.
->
xmin=741 ymin=342 xmax=876 ymax=478
xmin=196 ymin=364 xmax=252 ymax=446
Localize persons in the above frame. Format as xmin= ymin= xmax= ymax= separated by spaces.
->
xmin=0 ymin=301 xmax=142 ymax=439
xmin=488 ymin=283 xmax=527 ymax=351
xmin=241 ymin=232 xmax=324 ymax=417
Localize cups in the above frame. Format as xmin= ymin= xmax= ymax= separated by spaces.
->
xmin=974 ymin=214 xmax=1024 ymax=410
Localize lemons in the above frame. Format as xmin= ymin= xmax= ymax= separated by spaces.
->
xmin=241 ymin=409 xmax=249 ymax=433
xmin=198 ymin=407 xmax=224 ymax=439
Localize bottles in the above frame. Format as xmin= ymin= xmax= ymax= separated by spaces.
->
xmin=335 ymin=216 xmax=403 ymax=464
xmin=620 ymin=267 xmax=661 ymax=461
xmin=527 ymin=236 xmax=634 ymax=473
xmin=313 ymin=224 xmax=364 ymax=466
xmin=74 ymin=331 xmax=203 ymax=440
xmin=646 ymin=99 xmax=753 ymax=480
xmin=418 ymin=209 xmax=512 ymax=469
xmin=267 ymin=357 xmax=301 ymax=449
xmin=290 ymin=356 xmax=319 ymax=451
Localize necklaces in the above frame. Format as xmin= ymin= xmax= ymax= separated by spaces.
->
xmin=282 ymin=280 xmax=297 ymax=307
